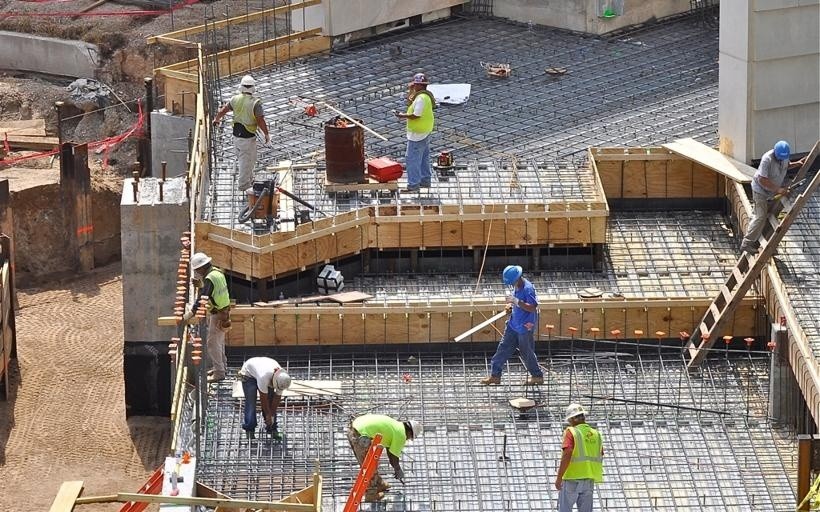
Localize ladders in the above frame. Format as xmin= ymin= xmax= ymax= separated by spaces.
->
xmin=682 ymin=140 xmax=820 ymax=372
xmin=342 ymin=433 xmax=384 ymax=512
xmin=118 ymin=463 xmax=164 ymax=512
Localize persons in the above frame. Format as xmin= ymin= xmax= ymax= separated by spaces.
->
xmin=212 ymin=74 xmax=270 ymax=191
xmin=480 ymin=265 xmax=544 ymax=385
xmin=395 ymin=73 xmax=436 ymax=192
xmin=182 ymin=251 xmax=231 ymax=384
xmin=739 ymin=139 xmax=808 ymax=253
xmin=237 ymin=356 xmax=291 ymax=439
xmin=556 ymin=403 xmax=604 ymax=512
xmin=347 ymin=412 xmax=421 ymax=502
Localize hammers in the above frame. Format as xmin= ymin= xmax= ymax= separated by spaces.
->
xmin=499 ymin=435 xmax=510 ymax=459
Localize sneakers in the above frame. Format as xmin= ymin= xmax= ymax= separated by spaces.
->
xmin=480 ymin=374 xmax=502 ymax=387
xmin=364 ymin=481 xmax=390 ymax=501
xmin=526 ymin=373 xmax=544 ymax=386
xmin=739 ymin=241 xmax=760 ymax=255
xmin=400 ymin=184 xmax=431 ymax=193
xmin=207 ymin=368 xmax=227 ymax=383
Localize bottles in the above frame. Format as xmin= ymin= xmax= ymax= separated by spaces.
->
xmin=175 ymin=448 xmax=181 ymax=462
xmin=183 ymin=451 xmax=189 ymax=463
xmin=172 ymin=471 xmax=178 ymax=490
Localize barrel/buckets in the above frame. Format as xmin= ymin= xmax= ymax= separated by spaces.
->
xmin=325 ymin=118 xmax=364 ymax=182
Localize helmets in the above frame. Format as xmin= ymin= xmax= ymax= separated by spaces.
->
xmin=240 ymin=74 xmax=257 ymax=87
xmin=501 ymin=264 xmax=524 ymax=285
xmin=565 ymin=402 xmax=587 ymax=420
xmin=412 ymin=72 xmax=430 ymax=86
xmin=190 ymin=251 xmax=213 ymax=272
xmin=773 ymin=139 xmax=790 ymax=160
xmin=272 ymin=368 xmax=293 ymax=395
xmin=409 ymin=418 xmax=422 ymax=440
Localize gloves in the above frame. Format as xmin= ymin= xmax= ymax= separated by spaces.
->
xmin=264 ymin=134 xmax=272 ymax=145
xmin=505 ymin=294 xmax=520 ymax=308
xmin=182 ymin=311 xmax=194 ymax=321
